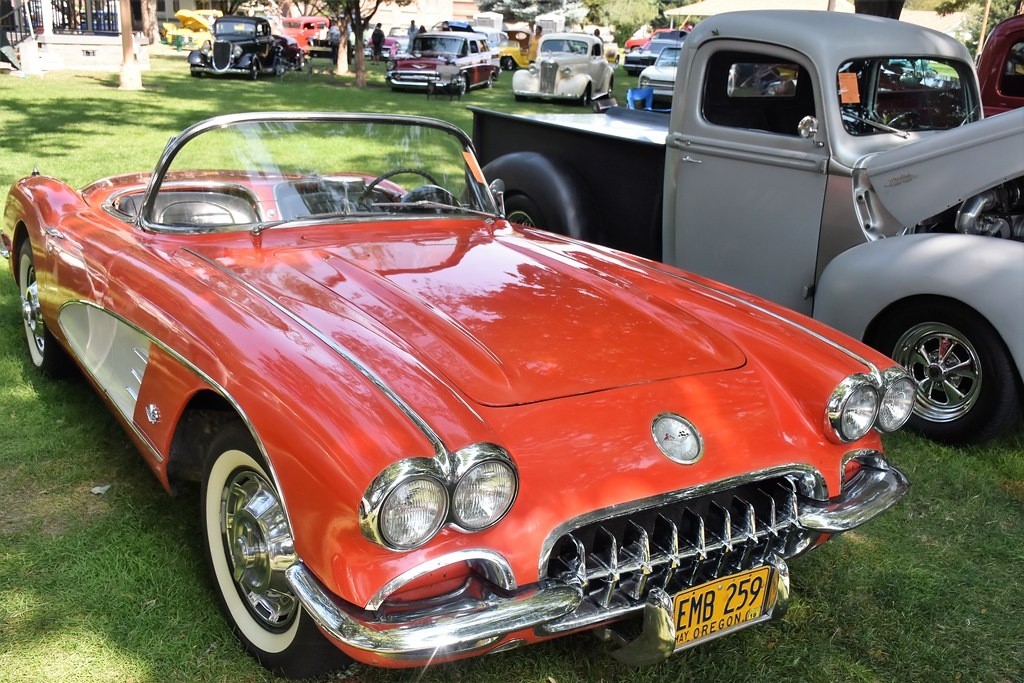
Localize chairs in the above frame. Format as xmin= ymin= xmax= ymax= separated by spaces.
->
xmin=274 ymin=177 xmax=395 ymax=220
xmin=114 ymin=192 xmax=259 ymax=225
xmin=629 ymin=88 xmax=669 ymax=114
xmin=427 ymin=64 xmax=461 ymax=101
xmin=223 ymin=23 xmax=234 ymax=30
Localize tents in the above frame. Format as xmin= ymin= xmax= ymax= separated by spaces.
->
xmin=664 ymin=0 xmax=968 ymax=126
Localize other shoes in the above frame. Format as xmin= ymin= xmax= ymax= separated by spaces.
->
xmin=371 ymin=62 xmax=375 ymax=64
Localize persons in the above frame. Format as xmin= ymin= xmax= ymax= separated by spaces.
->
xmin=527 ymin=26 xmax=544 ymax=64
xmin=208 ymin=9 xmax=219 ymax=39
xmin=329 ymin=20 xmax=452 ymax=73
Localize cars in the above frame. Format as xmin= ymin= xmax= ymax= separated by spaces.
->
xmin=24 ymin=10 xmax=117 ymax=36
xmin=638 ymin=42 xmax=735 ymax=107
xmin=512 ymin=33 xmax=614 ymax=107
xmin=975 ymin=13 xmax=1024 ymax=119
xmin=386 ymin=31 xmax=501 ymax=96
xmin=163 ymin=9 xmax=331 ymax=66
xmin=363 ymin=11 xmax=960 ymax=91
xmin=187 ymin=16 xmax=285 ymax=81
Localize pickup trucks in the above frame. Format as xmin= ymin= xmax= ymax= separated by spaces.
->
xmin=466 ymin=8 xmax=1024 ymax=449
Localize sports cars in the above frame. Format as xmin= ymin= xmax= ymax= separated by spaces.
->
xmin=0 ymin=114 xmax=920 ymax=677
